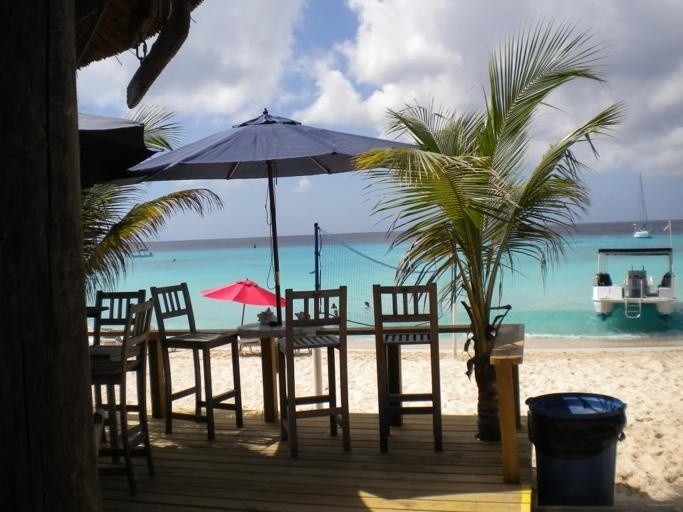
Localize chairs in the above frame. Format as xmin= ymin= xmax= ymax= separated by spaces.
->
xmin=91 ymin=298 xmax=157 ymax=497
xmin=275 ymin=284 xmax=352 ymax=457
xmin=371 ymin=281 xmax=445 ymax=454
xmin=148 ymin=282 xmax=242 ymax=443
xmin=93 ymin=288 xmax=145 ymax=463
xmin=85 ymin=304 xmax=108 ymax=346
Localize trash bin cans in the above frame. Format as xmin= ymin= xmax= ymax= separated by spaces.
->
xmin=525 ymin=392 xmax=627 ymax=506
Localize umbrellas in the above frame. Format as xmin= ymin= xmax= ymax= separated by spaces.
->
xmin=200 ymin=278 xmax=286 ymax=327
xmin=78 ymin=112 xmax=170 ymax=190
xmin=125 ymin=107 xmax=424 ymax=439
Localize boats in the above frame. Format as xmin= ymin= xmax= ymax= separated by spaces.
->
xmin=130 ymin=248 xmax=155 ymax=257
xmin=592 ymin=216 xmax=679 ymax=324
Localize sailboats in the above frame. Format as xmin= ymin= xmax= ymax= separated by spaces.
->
xmin=633 ymin=171 xmax=651 ymax=238
